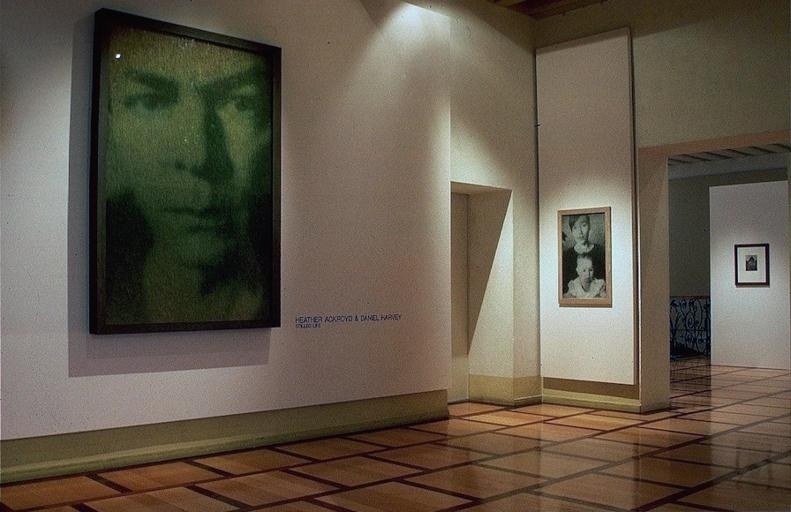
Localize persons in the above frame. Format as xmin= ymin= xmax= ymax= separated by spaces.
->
xmin=747 ymin=256 xmax=756 ymax=270
xmin=101 ymin=27 xmax=279 ymax=326
xmin=566 ymin=254 xmax=604 ymax=299
xmin=565 ymin=215 xmax=609 ymax=300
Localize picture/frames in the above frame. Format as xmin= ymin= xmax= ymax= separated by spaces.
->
xmin=86 ymin=8 xmax=284 ymax=339
xmin=555 ymin=206 xmax=613 ymax=308
xmin=734 ymin=243 xmax=770 ymax=286
